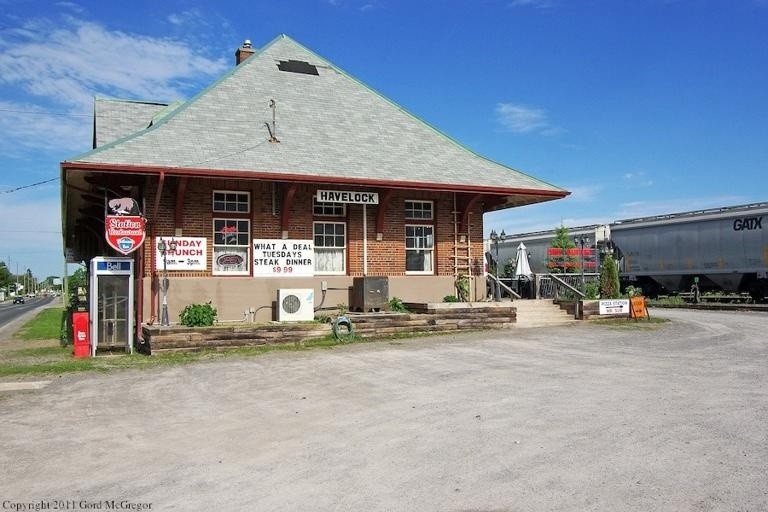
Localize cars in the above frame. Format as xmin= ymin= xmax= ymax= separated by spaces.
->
xmin=12 ymin=296 xmax=25 ymax=305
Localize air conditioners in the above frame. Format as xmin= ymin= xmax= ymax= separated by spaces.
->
xmin=275 ymin=288 xmax=315 ymax=321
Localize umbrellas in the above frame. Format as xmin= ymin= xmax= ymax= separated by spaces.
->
xmin=512 ymin=241 xmax=534 ymax=280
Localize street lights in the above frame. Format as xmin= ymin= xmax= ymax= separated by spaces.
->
xmin=574 ymin=231 xmax=591 ymax=294
xmin=490 ymin=229 xmax=508 ymax=276
